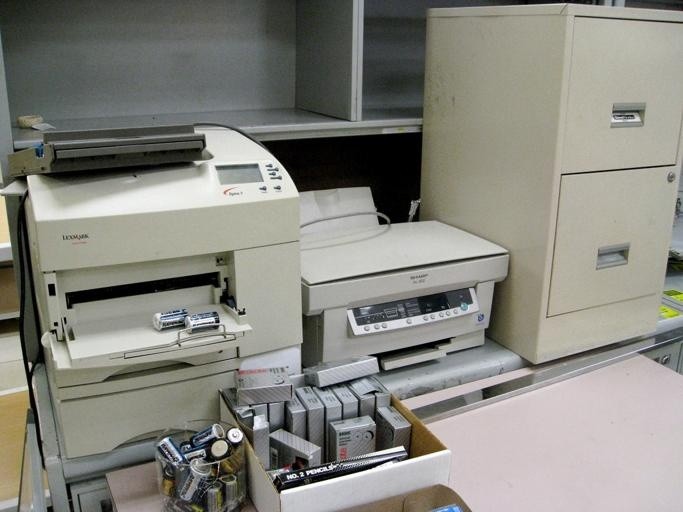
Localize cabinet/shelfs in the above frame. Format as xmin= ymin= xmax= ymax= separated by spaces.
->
xmin=421 ymin=3 xmax=683 ymax=366
xmin=0 ymin=0 xmax=420 ymax=196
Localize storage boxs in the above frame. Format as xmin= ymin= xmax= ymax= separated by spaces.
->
xmin=214 ymin=371 xmax=453 ymax=511
